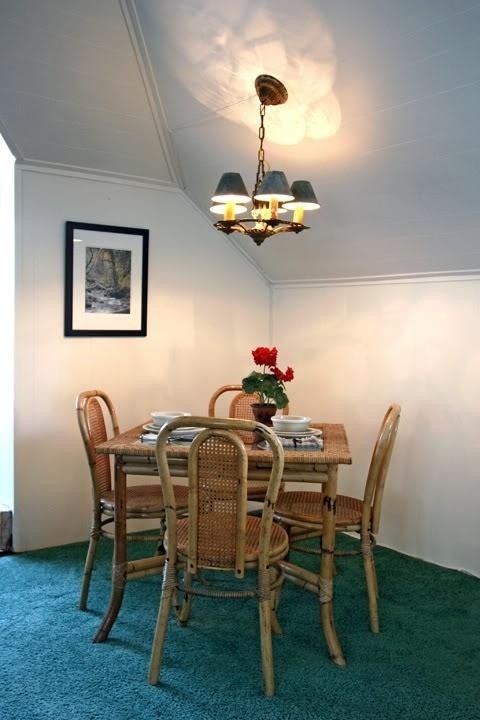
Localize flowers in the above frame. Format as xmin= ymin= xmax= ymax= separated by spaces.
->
xmin=241 ymin=347 xmax=294 ymax=409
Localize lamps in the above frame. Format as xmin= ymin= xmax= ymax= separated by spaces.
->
xmin=208 ymin=74 xmax=322 ymax=246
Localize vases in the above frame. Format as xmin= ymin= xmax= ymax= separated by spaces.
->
xmin=249 ymin=404 xmax=278 ymax=424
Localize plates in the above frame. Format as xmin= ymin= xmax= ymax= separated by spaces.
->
xmin=256 ymin=426 xmax=323 ymax=452
xmin=142 ymin=423 xmax=206 ymax=435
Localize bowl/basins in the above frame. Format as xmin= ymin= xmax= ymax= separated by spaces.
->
xmin=271 ymin=415 xmax=312 ymax=431
xmin=151 ymin=411 xmax=192 ymax=427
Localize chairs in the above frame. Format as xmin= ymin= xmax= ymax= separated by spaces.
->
xmin=149 ymin=414 xmax=290 ymax=699
xmin=76 ymin=389 xmax=191 ymax=611
xmin=272 ymin=405 xmax=401 ymax=667
xmin=208 ymin=384 xmax=288 ymax=582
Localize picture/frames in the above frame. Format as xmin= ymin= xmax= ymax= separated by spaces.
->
xmin=63 ymin=219 xmax=149 ymax=338
